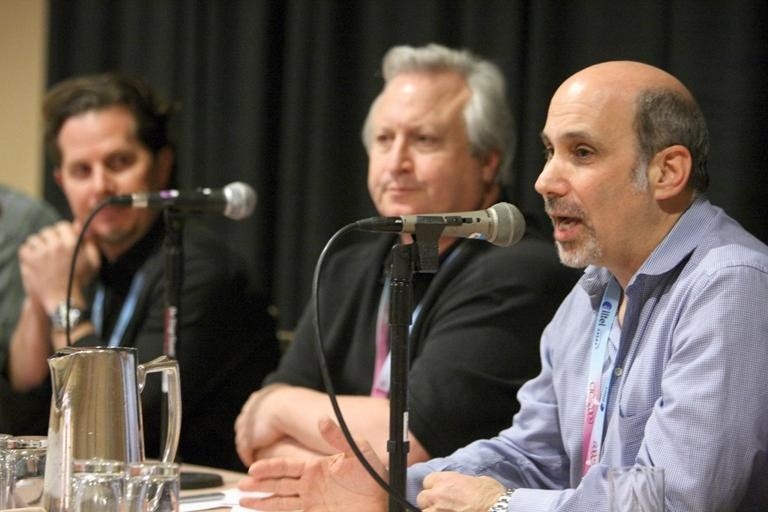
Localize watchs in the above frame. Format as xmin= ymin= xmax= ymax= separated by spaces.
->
xmin=50 ymin=299 xmax=90 ymax=334
xmin=489 ymin=487 xmax=518 ymax=512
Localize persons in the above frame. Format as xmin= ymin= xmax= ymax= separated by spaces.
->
xmin=238 ymin=60 xmax=768 ymax=512
xmin=0 ymin=186 xmax=61 ymax=436
xmin=233 ymin=43 xmax=588 ymax=475
xmin=0 ymin=74 xmax=280 ymax=472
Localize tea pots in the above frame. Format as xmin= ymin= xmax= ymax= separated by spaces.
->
xmin=42 ymin=345 xmax=184 ymax=512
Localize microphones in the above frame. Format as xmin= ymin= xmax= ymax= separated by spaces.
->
xmin=357 ymin=200 xmax=526 ymax=248
xmin=109 ymin=180 xmax=258 ymax=222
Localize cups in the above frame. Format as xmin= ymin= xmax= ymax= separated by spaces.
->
xmin=67 ymin=456 xmax=182 ymax=512
xmin=0 ymin=432 xmax=49 ymax=512
